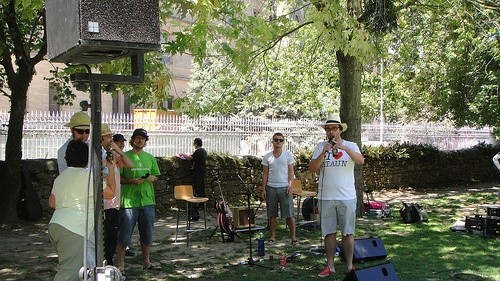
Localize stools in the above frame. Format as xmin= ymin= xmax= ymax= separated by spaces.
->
xmin=173 ymin=186 xmax=209 ymax=245
xmin=285 ymin=180 xmax=316 ymax=234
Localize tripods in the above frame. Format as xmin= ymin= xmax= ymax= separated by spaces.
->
xmin=222 ymin=173 xmax=273 ymax=269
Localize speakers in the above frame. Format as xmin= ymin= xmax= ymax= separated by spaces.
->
xmin=341 ymin=237 xmax=388 ymax=262
xmin=342 ymin=260 xmax=400 ymax=281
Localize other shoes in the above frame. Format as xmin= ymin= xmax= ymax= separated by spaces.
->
xmin=268 ymin=239 xmax=275 ymax=243
xmin=318 ymin=266 xmax=336 ymax=277
xmin=292 ymin=240 xmax=299 ymax=243
xmin=121 ymin=275 xmax=127 ymax=281
xmin=125 ymin=250 xmax=135 ymax=256
xmin=346 ymin=264 xmax=355 ymax=274
xmin=143 ymin=263 xmax=163 ymax=270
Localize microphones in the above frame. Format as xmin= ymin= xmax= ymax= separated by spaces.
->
xmin=326 ymin=136 xmax=336 ymax=152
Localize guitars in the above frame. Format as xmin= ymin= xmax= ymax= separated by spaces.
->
xmin=210 ymin=170 xmax=235 ymax=235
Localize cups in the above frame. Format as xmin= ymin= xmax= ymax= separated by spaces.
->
xmin=270 ymin=255 xmax=273 ymax=261
xmin=279 ymin=255 xmax=287 ymax=267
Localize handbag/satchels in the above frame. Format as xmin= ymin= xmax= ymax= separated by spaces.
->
xmin=400 ymin=203 xmax=420 ymax=223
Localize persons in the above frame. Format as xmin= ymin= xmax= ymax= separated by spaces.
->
xmin=117 ymin=128 xmax=163 ymax=281
xmin=112 ymin=133 xmax=127 ymax=151
xmin=180 ymin=137 xmax=208 ymax=222
xmin=99 ymin=124 xmax=135 ymax=267
xmin=49 ymin=141 xmax=115 ymax=281
xmin=57 ymin=111 xmax=111 ymax=267
xmin=260 ymin=132 xmax=298 ymax=244
xmin=309 ymin=115 xmax=365 ymax=277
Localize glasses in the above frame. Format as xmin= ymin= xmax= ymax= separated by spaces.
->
xmin=73 ymin=127 xmax=90 ymax=134
xmin=324 ymin=127 xmax=340 ymax=131
xmin=273 ymin=138 xmax=284 ymax=142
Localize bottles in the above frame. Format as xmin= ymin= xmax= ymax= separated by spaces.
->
xmin=257 ymin=239 xmax=265 ymax=257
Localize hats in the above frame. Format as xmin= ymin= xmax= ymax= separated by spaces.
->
xmin=64 ymin=112 xmax=91 ymax=129
xmin=132 ymin=129 xmax=148 ymax=139
xmin=113 ymin=134 xmax=126 ymax=141
xmin=100 ymin=123 xmax=112 ymax=135
xmin=318 ymin=113 xmax=347 ymax=131
